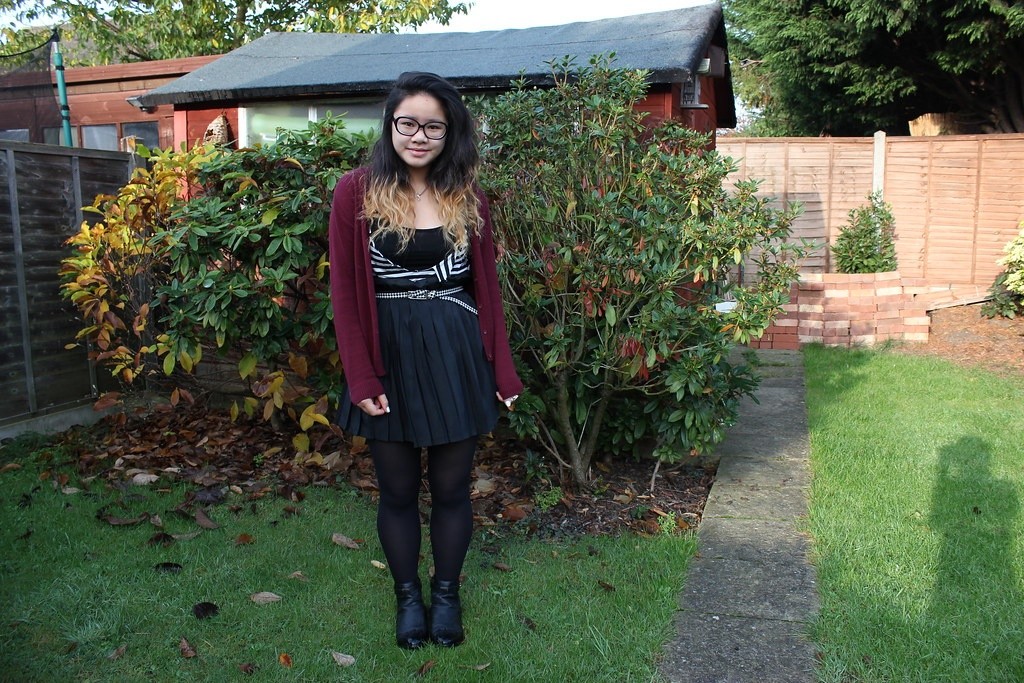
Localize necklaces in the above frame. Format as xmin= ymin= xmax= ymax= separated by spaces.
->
xmin=407 ymin=181 xmax=432 ymax=200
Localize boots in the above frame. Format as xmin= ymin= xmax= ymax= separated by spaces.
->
xmin=395 ymin=577 xmax=428 ymax=649
xmin=430 ymin=574 xmax=465 ymax=646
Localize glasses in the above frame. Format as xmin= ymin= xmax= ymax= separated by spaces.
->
xmin=391 ymin=116 xmax=449 ymax=140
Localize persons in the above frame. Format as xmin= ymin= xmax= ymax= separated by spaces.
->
xmin=326 ymin=69 xmax=526 ymax=649
